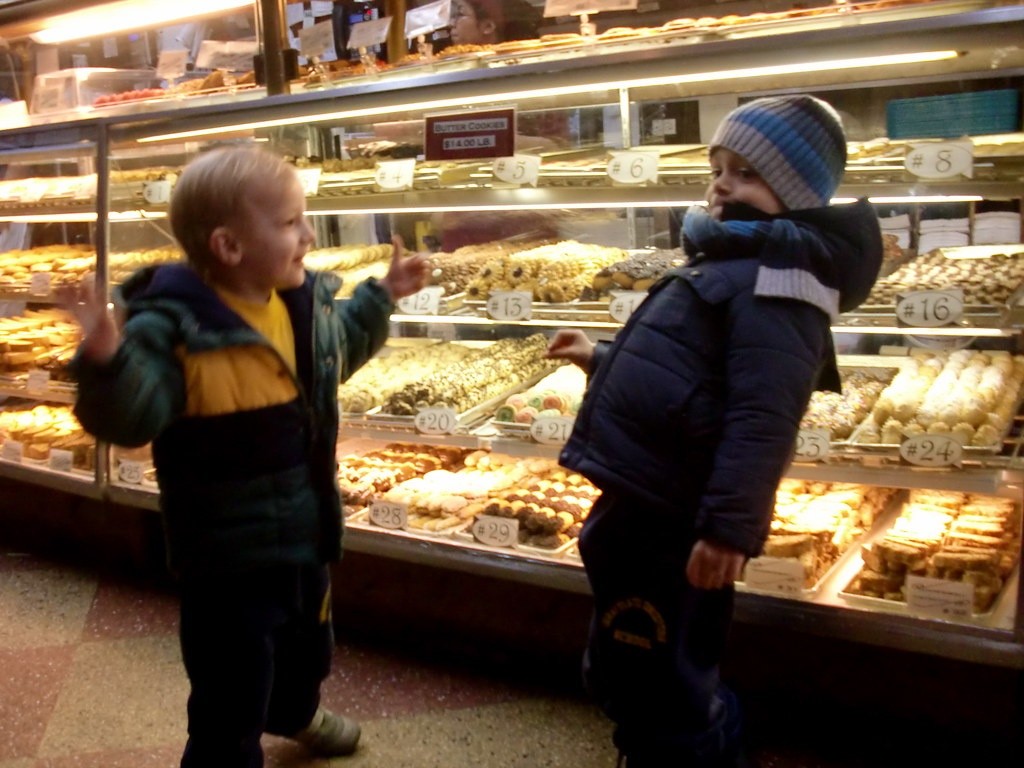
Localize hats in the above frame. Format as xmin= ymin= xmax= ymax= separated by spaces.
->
xmin=707 ymin=94 xmax=847 ymax=210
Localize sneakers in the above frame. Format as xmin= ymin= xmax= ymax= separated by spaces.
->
xmin=292 ymin=703 xmax=360 ymax=754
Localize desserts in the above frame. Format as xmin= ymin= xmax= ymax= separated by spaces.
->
xmin=0 ymin=1 xmax=1024 ymax=615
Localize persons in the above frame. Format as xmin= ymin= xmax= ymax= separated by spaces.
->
xmin=450 ymin=0 xmax=540 ymax=44
xmin=52 ymin=144 xmax=433 ymax=768
xmin=540 ymin=96 xmax=880 ymax=768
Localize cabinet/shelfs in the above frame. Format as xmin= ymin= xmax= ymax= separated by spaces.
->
xmin=0 ymin=0 xmax=1024 ymax=768
